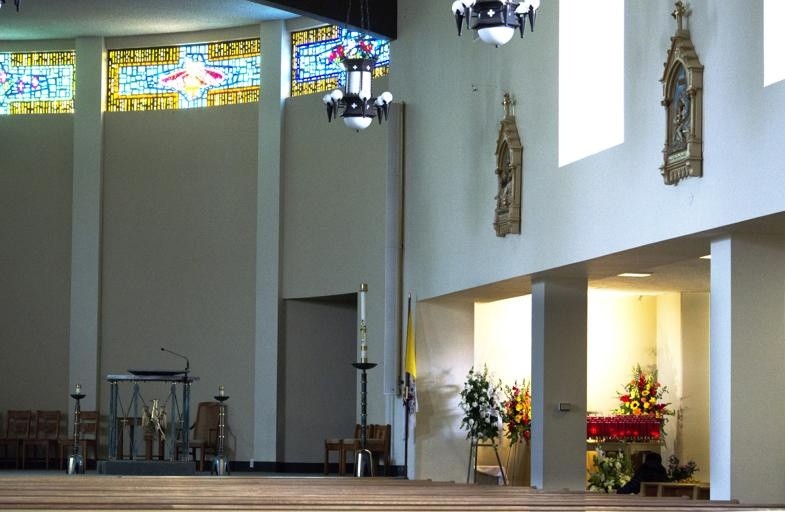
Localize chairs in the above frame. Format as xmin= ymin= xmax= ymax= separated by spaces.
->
xmin=175 ymin=402 xmax=231 ymax=475
xmin=0 ymin=408 xmax=100 ymax=471
xmin=323 ymin=423 xmax=391 ymax=476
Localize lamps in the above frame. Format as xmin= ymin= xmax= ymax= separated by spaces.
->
xmin=322 ymin=1 xmax=392 ymax=133
xmin=451 ymin=0 xmax=541 ymax=50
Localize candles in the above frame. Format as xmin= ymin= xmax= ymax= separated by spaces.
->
xmin=74 ymin=383 xmax=80 ymax=395
xmin=219 ymin=384 xmax=224 ymax=396
xmin=359 ymin=282 xmax=369 ymax=364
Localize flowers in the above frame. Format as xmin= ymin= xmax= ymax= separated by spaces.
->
xmin=457 ymin=361 xmax=532 ymax=443
xmin=584 ymin=440 xmax=634 ymax=494
xmin=611 ymin=361 xmax=676 ymax=418
